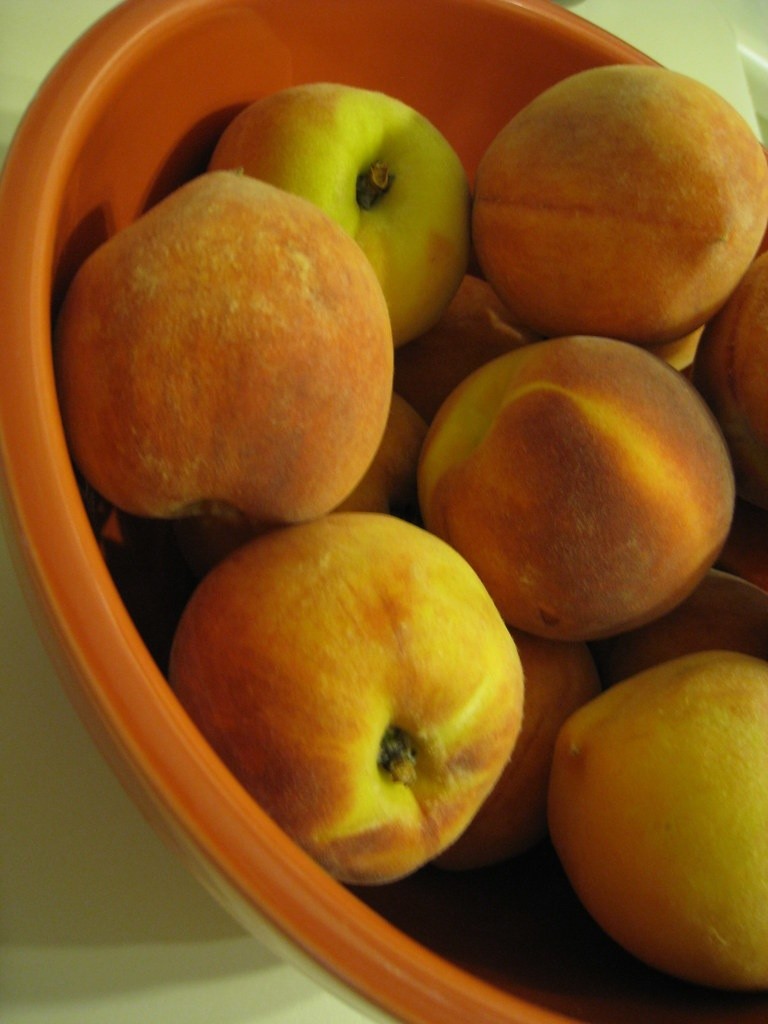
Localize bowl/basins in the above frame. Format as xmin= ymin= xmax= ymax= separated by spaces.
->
xmin=1 ymin=0 xmax=768 ymax=1024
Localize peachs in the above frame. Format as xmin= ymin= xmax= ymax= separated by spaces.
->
xmin=57 ymin=55 xmax=768 ymax=1008
